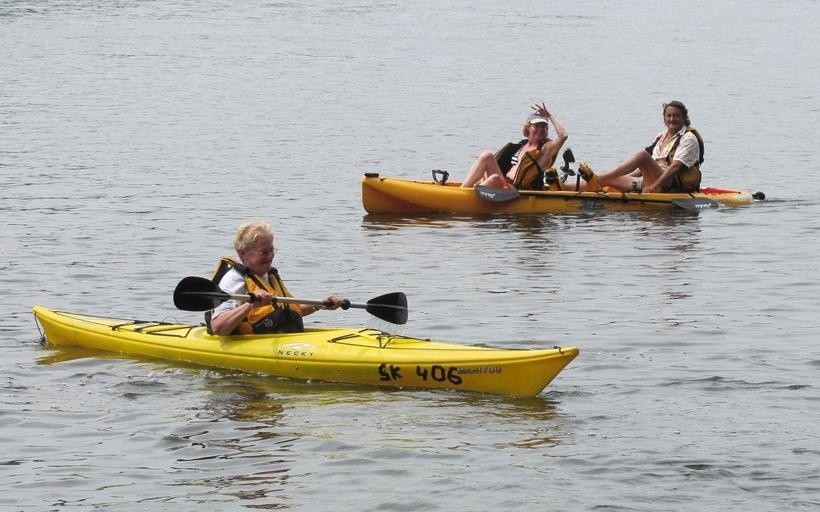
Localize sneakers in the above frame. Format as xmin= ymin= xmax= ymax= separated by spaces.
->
xmin=547 ymin=167 xmax=563 ymax=191
xmin=581 ymin=161 xmax=602 ymax=193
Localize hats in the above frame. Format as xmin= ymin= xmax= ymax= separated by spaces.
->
xmin=527 ymin=113 xmax=548 ymax=125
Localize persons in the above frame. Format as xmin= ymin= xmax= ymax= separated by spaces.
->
xmin=542 ymin=99 xmax=706 ymax=195
xmin=457 ymin=101 xmax=569 ymax=192
xmin=205 ymin=222 xmax=351 ymax=337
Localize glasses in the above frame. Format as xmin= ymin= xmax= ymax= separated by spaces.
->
xmin=249 ymin=247 xmax=278 ymax=258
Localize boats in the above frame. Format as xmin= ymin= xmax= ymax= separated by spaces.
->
xmin=357 ymin=172 xmax=757 ymax=223
xmin=29 ymin=306 xmax=580 ymax=405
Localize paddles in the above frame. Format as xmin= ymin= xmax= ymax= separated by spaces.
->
xmin=474 ymin=184 xmax=718 ymax=210
xmin=173 ymin=276 xmax=408 ymax=324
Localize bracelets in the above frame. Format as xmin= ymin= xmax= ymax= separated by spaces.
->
xmin=311 ymin=300 xmax=324 ymax=313
